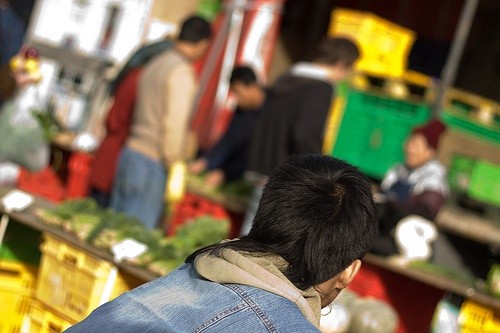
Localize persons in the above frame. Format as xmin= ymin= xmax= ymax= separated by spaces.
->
xmin=61 ymin=155 xmax=379 ymax=333
xmin=381 ymin=118 xmax=448 ymax=259
xmin=238 ymin=39 xmax=360 ymax=237
xmin=76 ymin=62 xmax=115 ymax=134
xmin=187 ymin=67 xmax=265 ymax=198
xmin=0 ymin=48 xmax=50 ymax=169
xmin=87 ymin=15 xmax=214 ymax=226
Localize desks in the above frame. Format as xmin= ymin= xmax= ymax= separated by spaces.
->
xmin=0 ymin=183 xmax=166 ymax=283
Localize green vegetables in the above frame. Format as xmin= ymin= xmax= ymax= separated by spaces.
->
xmin=45 ymin=197 xmax=231 ymax=274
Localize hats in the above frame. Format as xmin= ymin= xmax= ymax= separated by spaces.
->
xmin=414 ymin=120 xmax=446 ymax=149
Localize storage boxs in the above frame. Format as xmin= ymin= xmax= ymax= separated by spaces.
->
xmin=0 ymin=232 xmax=136 ymax=333
xmin=329 ymin=8 xmax=415 ymax=78
